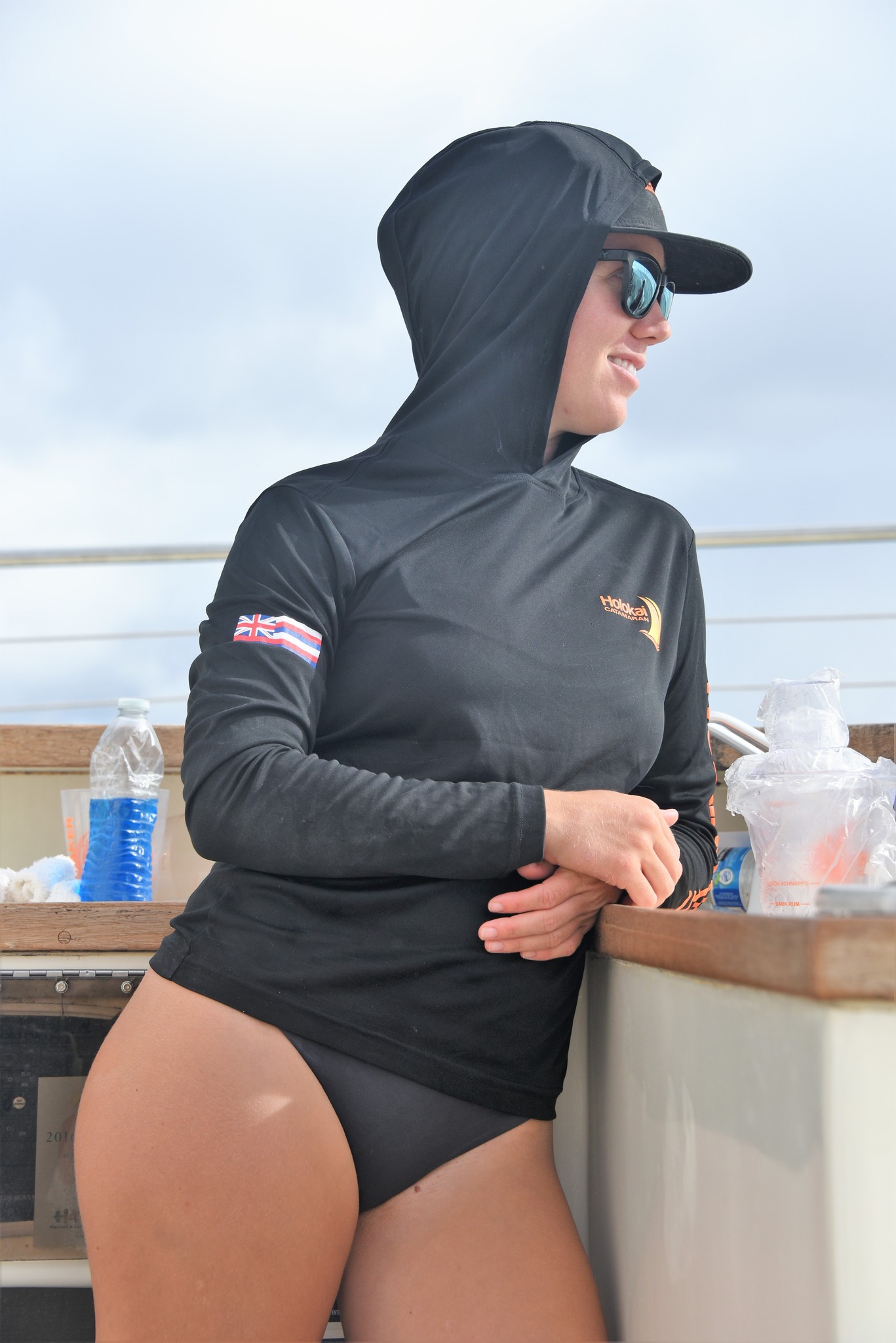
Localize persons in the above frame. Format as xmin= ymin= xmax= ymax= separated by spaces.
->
xmin=66 ymin=113 xmax=730 ymax=1343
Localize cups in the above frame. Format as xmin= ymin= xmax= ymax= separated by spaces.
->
xmin=147 ymin=788 xmax=169 ymax=902
xmin=62 ymin=789 xmax=91 ymax=881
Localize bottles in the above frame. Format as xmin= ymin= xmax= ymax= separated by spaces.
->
xmin=705 ymin=847 xmax=757 ymax=913
xmin=78 ymin=698 xmax=163 ymax=901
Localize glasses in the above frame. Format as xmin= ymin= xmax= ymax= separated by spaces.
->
xmin=596 ymin=248 xmax=676 ymax=320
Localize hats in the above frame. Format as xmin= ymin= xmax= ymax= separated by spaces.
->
xmin=606 ymin=178 xmax=753 ymax=295
